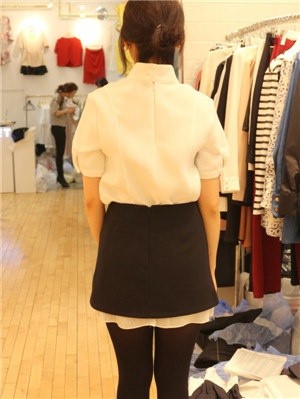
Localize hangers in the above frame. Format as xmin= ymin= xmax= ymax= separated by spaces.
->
xmin=219 ymin=13 xmax=300 ymax=40
xmin=24 ymin=95 xmax=53 ymax=110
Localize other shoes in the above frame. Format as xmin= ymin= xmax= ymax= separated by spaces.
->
xmin=57 ymin=176 xmax=68 ymax=187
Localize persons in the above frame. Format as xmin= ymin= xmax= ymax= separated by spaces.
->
xmin=49 ymin=83 xmax=77 ymax=187
xmin=95 ymin=78 xmax=107 ymax=89
xmin=71 ymin=0 xmax=231 ymax=399
